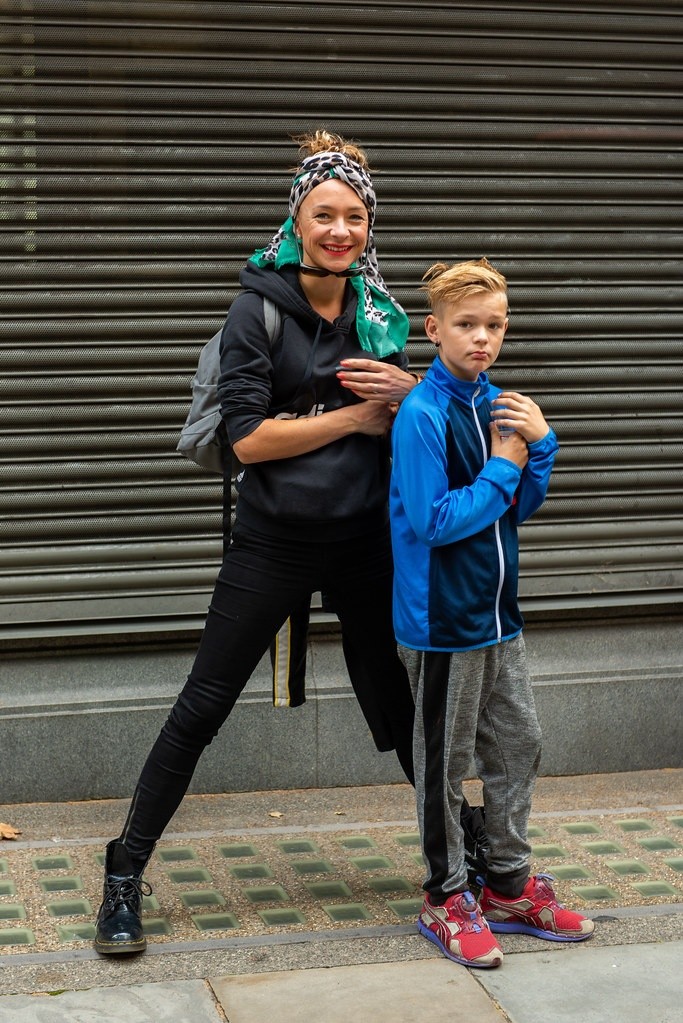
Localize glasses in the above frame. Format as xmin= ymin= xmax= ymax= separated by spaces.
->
xmin=293 ymin=217 xmax=368 ymax=278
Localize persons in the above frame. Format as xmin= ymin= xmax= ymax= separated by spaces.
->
xmin=93 ymin=133 xmax=487 ymax=957
xmin=389 ymin=255 xmax=597 ymax=971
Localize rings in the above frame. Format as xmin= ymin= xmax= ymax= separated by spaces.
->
xmin=373 ymin=385 xmax=379 ymax=394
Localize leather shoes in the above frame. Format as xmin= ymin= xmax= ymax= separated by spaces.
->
xmin=463 ymin=806 xmax=486 ymax=891
xmin=94 ymin=838 xmax=146 ymax=953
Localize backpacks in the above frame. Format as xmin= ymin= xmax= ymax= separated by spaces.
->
xmin=176 ymin=296 xmax=283 ymax=479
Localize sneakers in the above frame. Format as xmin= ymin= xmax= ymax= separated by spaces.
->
xmin=478 ymin=873 xmax=595 ymax=942
xmin=417 ymin=884 xmax=503 ymax=968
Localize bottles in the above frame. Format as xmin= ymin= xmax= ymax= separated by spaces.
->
xmin=492 ymin=404 xmax=517 ymax=505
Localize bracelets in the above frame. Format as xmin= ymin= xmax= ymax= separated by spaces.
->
xmin=396 ymin=373 xmax=420 ymax=405
